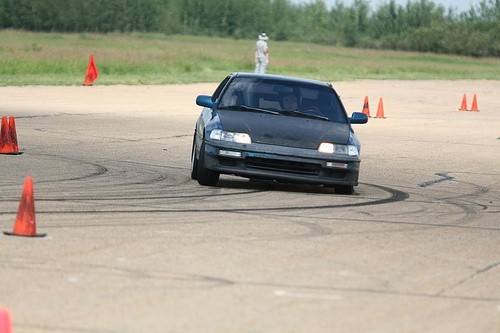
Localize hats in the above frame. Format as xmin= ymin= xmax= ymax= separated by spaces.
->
xmin=258 ymin=33 xmax=268 ymax=40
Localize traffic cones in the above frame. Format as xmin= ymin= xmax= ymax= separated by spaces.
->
xmin=8 ymin=116 xmax=24 ymax=155
xmin=2 ymin=175 xmax=47 ymax=238
xmin=0 ymin=308 xmax=13 ymax=333
xmin=82 ymin=54 xmax=99 ymax=86
xmin=459 ymin=93 xmax=468 ymax=111
xmin=469 ymin=94 xmax=480 ymax=112
xmin=373 ymin=97 xmax=386 ymax=118
xmin=0 ymin=115 xmax=18 ymax=155
xmin=362 ymin=95 xmax=371 ymax=118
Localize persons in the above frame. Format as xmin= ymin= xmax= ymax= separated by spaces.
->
xmin=279 ymin=86 xmax=302 ymax=111
xmin=255 ymin=33 xmax=269 ymax=74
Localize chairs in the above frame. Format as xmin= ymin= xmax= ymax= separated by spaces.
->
xmin=236 ymin=93 xmax=258 ymax=108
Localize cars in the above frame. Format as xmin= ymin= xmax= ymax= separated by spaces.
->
xmin=190 ymin=73 xmax=368 ymax=194
